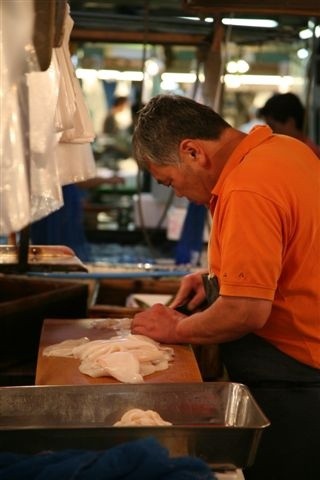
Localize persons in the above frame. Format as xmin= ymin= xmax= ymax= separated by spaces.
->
xmin=235 ymin=106 xmax=264 ymax=132
xmin=33 ymin=175 xmax=125 ymax=261
xmin=128 ymin=102 xmax=145 ymax=132
xmin=103 ymin=96 xmax=126 ymax=134
xmin=131 ymin=95 xmax=320 ymax=480
xmin=257 ymin=92 xmax=319 ymax=158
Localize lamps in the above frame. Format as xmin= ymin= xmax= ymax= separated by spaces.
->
xmin=145 ymin=57 xmax=165 ymax=79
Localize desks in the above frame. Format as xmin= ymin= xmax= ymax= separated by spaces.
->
xmin=34 ymin=317 xmax=204 ymax=385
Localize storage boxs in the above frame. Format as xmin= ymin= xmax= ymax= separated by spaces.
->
xmin=1 ymin=382 xmax=270 ymax=468
